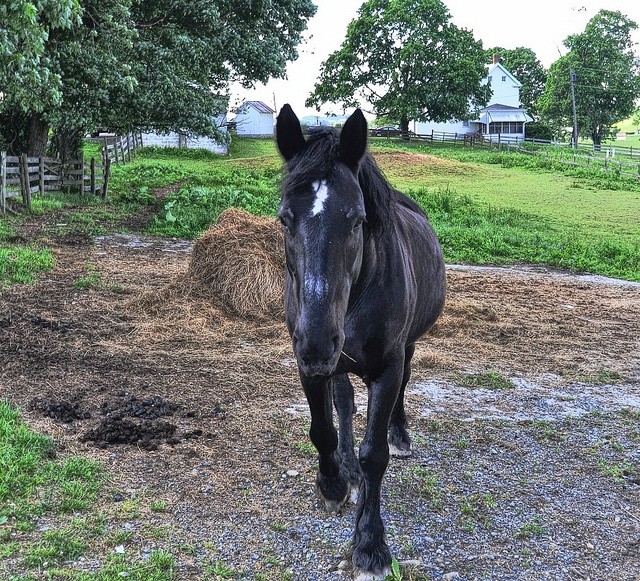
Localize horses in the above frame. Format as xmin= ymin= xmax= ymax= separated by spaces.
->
xmin=274 ymin=103 xmax=445 ymax=581
